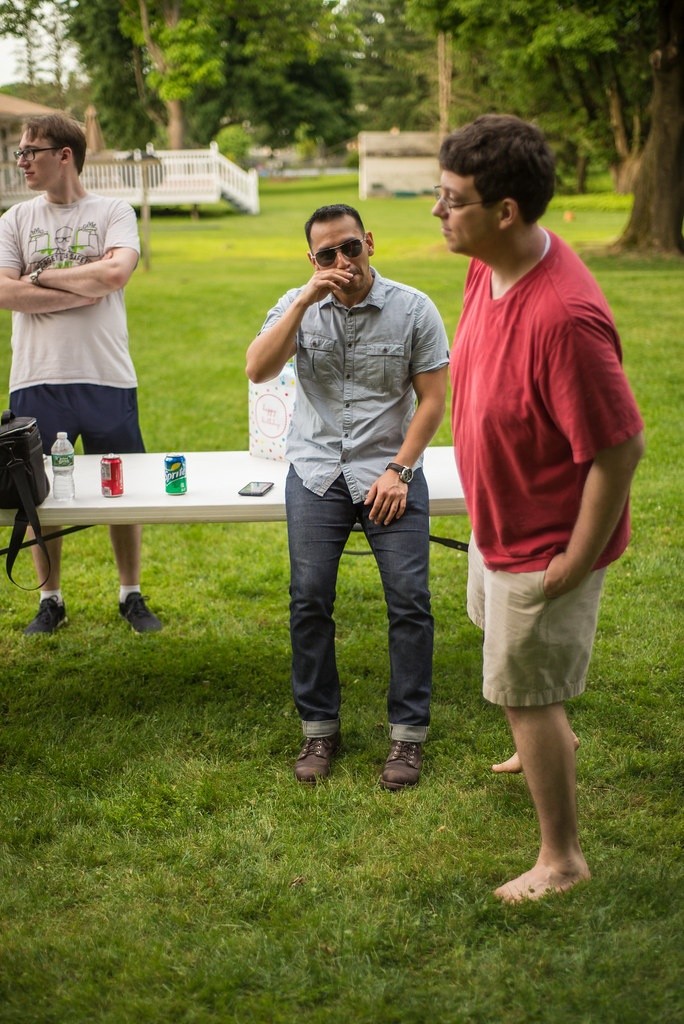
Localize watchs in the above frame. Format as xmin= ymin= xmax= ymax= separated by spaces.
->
xmin=385 ymin=461 xmax=414 ymax=483
xmin=29 ymin=268 xmax=44 ymax=289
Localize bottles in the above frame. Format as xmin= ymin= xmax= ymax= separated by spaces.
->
xmin=51 ymin=431 xmax=75 ymax=500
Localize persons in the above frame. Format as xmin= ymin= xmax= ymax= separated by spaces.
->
xmin=0 ymin=113 xmax=160 ymax=636
xmin=432 ymin=113 xmax=646 ymax=906
xmin=245 ymin=203 xmax=450 ymax=790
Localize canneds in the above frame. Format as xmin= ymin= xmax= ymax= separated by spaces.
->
xmin=100 ymin=453 xmax=124 ymax=497
xmin=164 ymin=454 xmax=187 ymax=495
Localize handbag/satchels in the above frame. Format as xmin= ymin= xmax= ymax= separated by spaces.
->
xmin=0 ymin=409 xmax=51 ymax=590
xmin=248 ymin=363 xmax=297 ymax=463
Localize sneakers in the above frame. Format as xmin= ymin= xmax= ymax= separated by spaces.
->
xmin=378 ymin=739 xmax=426 ymax=789
xmin=117 ymin=592 xmax=162 ymax=636
xmin=22 ymin=595 xmax=68 ymax=635
xmin=294 ymin=730 xmax=343 ymax=784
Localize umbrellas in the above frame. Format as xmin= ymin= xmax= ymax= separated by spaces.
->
xmin=0 ymin=93 xmax=109 ymax=155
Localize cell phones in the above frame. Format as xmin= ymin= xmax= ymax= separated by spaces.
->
xmin=239 ymin=482 xmax=273 ymax=496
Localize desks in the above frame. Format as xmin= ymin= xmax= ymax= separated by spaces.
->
xmin=0 ymin=446 xmax=470 ymax=555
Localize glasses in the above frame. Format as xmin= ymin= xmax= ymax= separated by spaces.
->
xmin=431 ymin=185 xmax=483 ymax=214
xmin=13 ymin=147 xmax=63 ymax=160
xmin=309 ymin=238 xmax=367 ymax=268
